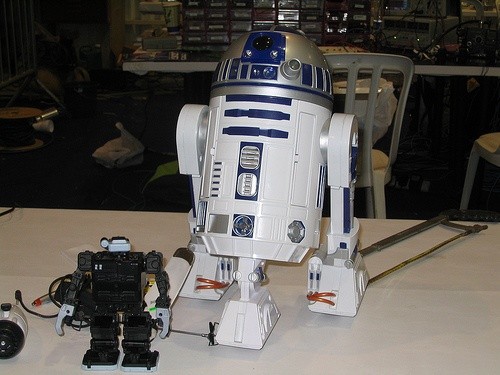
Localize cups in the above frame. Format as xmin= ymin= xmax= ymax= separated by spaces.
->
xmin=32 ymin=120 xmax=56 ymax=134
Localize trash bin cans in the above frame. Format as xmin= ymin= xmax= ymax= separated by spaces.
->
xmin=60 ymin=81 xmax=100 ymax=118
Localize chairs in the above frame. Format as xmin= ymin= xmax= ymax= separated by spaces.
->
xmin=324 ymin=52 xmax=415 ymax=218
xmin=459 ymin=131 xmax=500 ymax=210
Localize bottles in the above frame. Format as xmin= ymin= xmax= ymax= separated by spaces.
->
xmin=35 ymin=108 xmax=59 ymax=121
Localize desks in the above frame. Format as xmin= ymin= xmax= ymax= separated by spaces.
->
xmin=0 ymin=204 xmax=500 ymax=375
xmin=120 ymin=46 xmax=500 ymax=164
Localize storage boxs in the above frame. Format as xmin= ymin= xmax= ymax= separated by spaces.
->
xmin=207 ymin=32 xmax=230 ymax=44
xmin=300 ymin=22 xmax=323 ymax=34
xmin=231 ymin=8 xmax=252 ymax=21
xmin=349 ymin=12 xmax=371 ymax=22
xmin=231 ymin=32 xmax=243 ymax=41
xmin=184 ymin=32 xmax=206 ymax=44
xmin=207 ymin=20 xmax=229 ymax=33
xmin=324 ymin=22 xmax=348 ymax=34
xmin=207 ymin=8 xmax=228 ymax=19
xmin=230 ymin=21 xmax=252 ymax=32
xmin=305 ymin=34 xmax=369 ymax=47
xmin=183 ymin=20 xmax=206 ymax=32
xmin=252 ymin=21 xmax=276 ymax=31
xmin=277 ymin=21 xmax=300 ymax=31
xmin=301 ymin=10 xmax=323 ymax=21
xmin=277 ymin=9 xmax=300 ymax=21
xmin=349 ymin=22 xmax=370 ymax=32
xmin=324 ymin=11 xmax=347 ymax=22
xmin=182 ymin=9 xmax=206 ymax=19
xmin=182 ymin=0 xmax=371 ymax=11
xmin=252 ymin=8 xmax=276 ymax=21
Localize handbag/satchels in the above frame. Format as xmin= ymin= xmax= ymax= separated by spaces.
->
xmin=93 ymin=121 xmax=145 ymax=168
